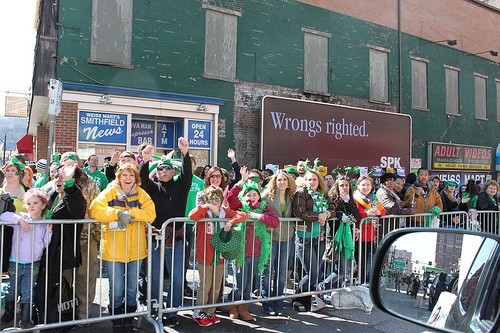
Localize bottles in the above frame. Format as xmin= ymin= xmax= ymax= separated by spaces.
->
xmin=410 ymin=194 xmax=416 ymax=208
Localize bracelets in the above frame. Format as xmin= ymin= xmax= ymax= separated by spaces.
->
xmin=325 ymin=211 xmax=328 ymax=220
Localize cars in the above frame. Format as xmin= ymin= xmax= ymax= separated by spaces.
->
xmin=429 ymin=274 xmax=459 ymax=311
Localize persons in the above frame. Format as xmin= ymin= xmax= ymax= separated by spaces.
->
xmin=228 ymin=166 xmax=280 ymax=320
xmin=0 ymin=137 xmax=394 ymax=328
xmin=0 ymin=188 xmax=53 ymax=330
xmin=395 ymin=268 xmax=448 ymax=312
xmin=188 ymin=185 xmax=248 ymax=327
xmin=476 ymin=180 xmax=500 ymax=235
xmin=352 ymin=174 xmax=387 ymax=286
xmin=396 ymin=173 xmax=500 ymax=232
xmin=404 ymin=167 xmax=443 ymax=227
xmin=88 ymin=162 xmax=156 ymax=333
xmin=292 ymin=169 xmax=336 ymax=311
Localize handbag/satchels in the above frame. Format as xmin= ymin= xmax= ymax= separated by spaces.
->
xmin=409 ymin=286 xmax=413 ymax=292
xmin=322 ymin=241 xmax=339 ymax=263
xmin=432 ymin=215 xmax=440 ymax=228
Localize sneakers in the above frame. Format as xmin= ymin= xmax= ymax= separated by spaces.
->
xmin=207 ymin=313 xmax=220 ymax=323
xmin=192 ymin=311 xmax=212 ymax=326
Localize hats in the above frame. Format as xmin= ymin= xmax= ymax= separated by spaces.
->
xmin=120 ymin=151 xmax=135 ymax=159
xmin=210 ymin=226 xmax=242 ymax=261
xmin=60 ymin=152 xmax=77 ymax=162
xmin=380 ymin=173 xmax=394 ymax=183
xmin=444 ymin=180 xmax=458 ymax=188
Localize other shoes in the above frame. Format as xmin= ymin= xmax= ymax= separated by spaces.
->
xmin=278 ymin=312 xmax=283 ymax=315
xmin=267 ymin=311 xmax=276 ymax=315
xmin=395 ymin=291 xmax=417 ymax=299
xmin=170 ymin=318 xmax=183 ymax=327
xmin=293 ymin=302 xmax=306 ymax=311
xmin=422 ymin=297 xmax=426 ymax=300
xmin=317 ymin=289 xmax=324 ymax=301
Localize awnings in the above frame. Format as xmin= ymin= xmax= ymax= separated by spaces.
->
xmin=16 ymin=134 xmax=33 ymax=153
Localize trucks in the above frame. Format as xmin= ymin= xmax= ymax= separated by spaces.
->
xmin=424 ymin=267 xmax=445 ymax=294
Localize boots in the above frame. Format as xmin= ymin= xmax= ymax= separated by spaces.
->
xmin=229 ymin=305 xmax=239 ymax=319
xmin=237 ymin=303 xmax=252 ymax=320
xmin=19 ymin=303 xmax=33 ymax=328
xmin=108 ymin=303 xmax=124 ymax=333
xmin=123 ymin=304 xmax=137 ymax=333
xmin=1 ymin=301 xmax=14 ymax=324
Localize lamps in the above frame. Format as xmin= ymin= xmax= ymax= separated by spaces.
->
xmin=466 ymin=50 xmax=498 ymax=57
xmin=198 ymin=104 xmax=208 ymax=111
xmin=100 ymin=96 xmax=105 ymax=102
xmin=106 ymin=96 xmax=112 ymax=104
xmin=426 ymin=39 xmax=458 ymax=46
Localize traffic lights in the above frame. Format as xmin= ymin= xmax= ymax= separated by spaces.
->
xmin=406 ymin=259 xmax=408 ymax=265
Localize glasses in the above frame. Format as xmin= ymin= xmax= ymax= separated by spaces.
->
xmin=157 ymin=165 xmax=173 ymax=171
xmin=211 ymin=175 xmax=222 ymax=178
xmin=54 ymin=174 xmax=60 ymax=178
xmin=248 ymin=177 xmax=261 ymax=181
